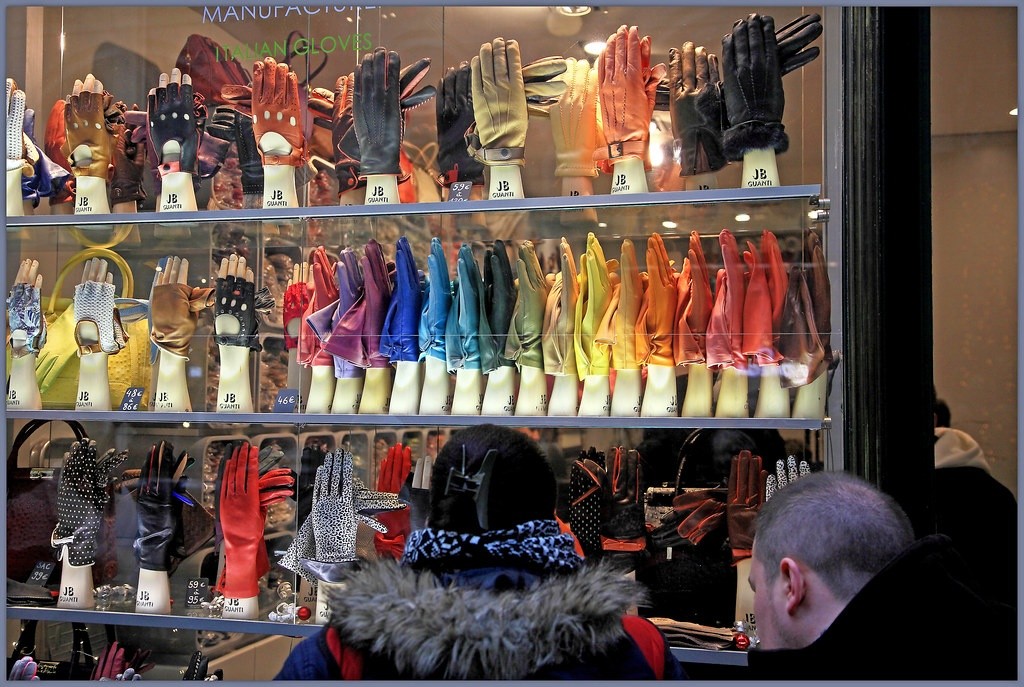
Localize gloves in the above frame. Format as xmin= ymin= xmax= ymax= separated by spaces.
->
xmin=48 ymin=437 xmax=411 ymax=599
xmin=205 ymin=99 xmax=266 ymax=194
xmin=8 ymin=641 xmax=224 ymax=682
xmin=125 ymin=92 xmax=230 ymax=195
xmin=308 ymin=12 xmax=823 ymax=218
xmin=569 ymin=442 xmax=815 ymax=567
xmin=5 ymin=77 xmax=148 ymax=206
xmin=297 ymin=230 xmax=835 ymax=390
xmin=220 ymin=57 xmax=309 ymax=169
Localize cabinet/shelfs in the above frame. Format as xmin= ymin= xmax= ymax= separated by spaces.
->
xmin=0 ymin=1 xmax=934 ymax=687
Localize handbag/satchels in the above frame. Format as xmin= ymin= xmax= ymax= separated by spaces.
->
xmin=636 ymin=428 xmax=824 ymax=629
xmin=174 ymin=30 xmax=448 ymax=204
xmin=7 ymin=420 xmax=118 ymax=591
xmin=6 ymin=248 xmax=154 ymax=411
xmin=6 ymin=619 xmax=97 ymax=681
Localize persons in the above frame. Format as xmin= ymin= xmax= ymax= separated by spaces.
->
xmin=744 ymin=384 xmax=1017 ymax=680
xmin=273 ymin=425 xmax=686 ymax=680
